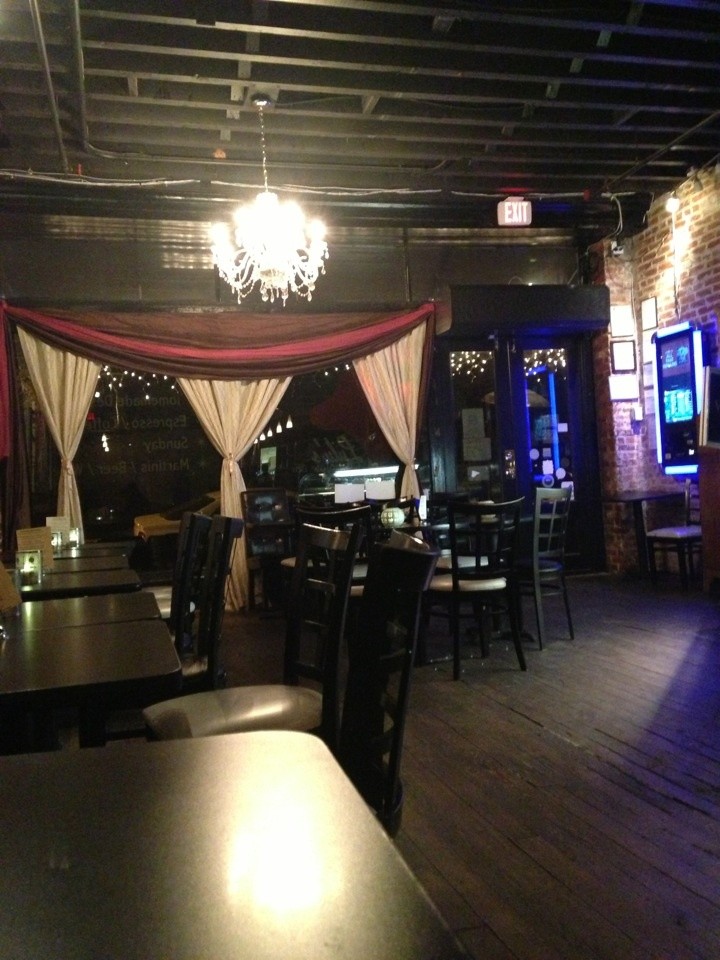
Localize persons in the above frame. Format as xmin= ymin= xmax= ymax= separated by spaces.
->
xmin=298 ymin=462 xmax=322 ymax=492
xmin=256 ymin=464 xmax=273 ymax=488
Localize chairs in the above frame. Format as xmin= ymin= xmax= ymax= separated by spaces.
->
xmin=645 ymin=479 xmax=703 ymax=592
xmin=78 ymin=486 xmax=574 ymax=838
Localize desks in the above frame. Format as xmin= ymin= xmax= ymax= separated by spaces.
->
xmin=589 ymin=486 xmax=686 ymax=580
xmin=0 ymin=729 xmax=471 ymax=960
xmin=0 ymin=540 xmax=183 ymax=754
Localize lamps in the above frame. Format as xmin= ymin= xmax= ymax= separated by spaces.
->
xmin=207 ymin=93 xmax=330 ymax=308
